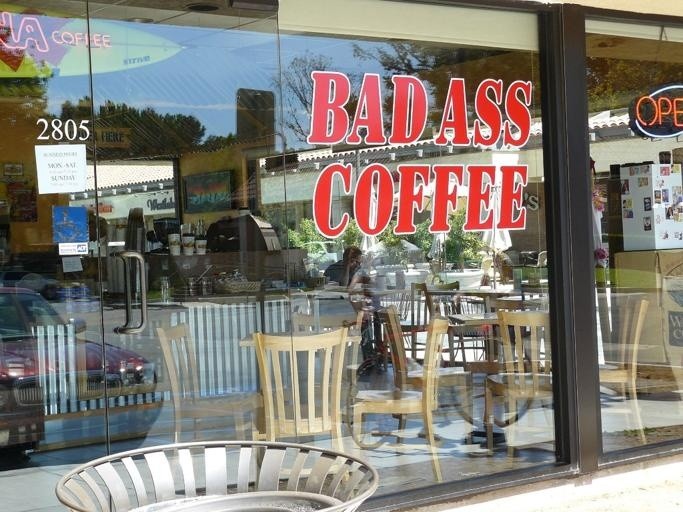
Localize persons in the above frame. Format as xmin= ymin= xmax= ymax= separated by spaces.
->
xmin=323 ymin=245 xmax=372 ymax=284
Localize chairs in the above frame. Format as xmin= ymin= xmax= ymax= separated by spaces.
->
xmin=598 ymin=299 xmax=647 ymax=445
xmin=155 ymin=280 xmax=549 ymax=481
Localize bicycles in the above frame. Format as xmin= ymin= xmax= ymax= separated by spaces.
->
xmin=345 ymin=284 xmax=536 ymax=449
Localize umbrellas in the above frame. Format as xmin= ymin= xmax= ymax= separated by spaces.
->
xmin=359 ymin=182 xmax=380 ymax=251
xmin=480 ymin=186 xmax=512 ymax=291
xmin=391 ymin=178 xmax=470 ymax=200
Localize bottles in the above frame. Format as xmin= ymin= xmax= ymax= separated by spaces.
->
xmin=196 ymin=218 xmax=207 ymax=240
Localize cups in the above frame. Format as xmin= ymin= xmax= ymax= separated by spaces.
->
xmin=168 ymin=234 xmax=207 ymax=257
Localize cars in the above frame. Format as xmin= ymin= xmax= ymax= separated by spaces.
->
xmin=300 ymin=239 xmax=345 ymax=277
xmin=0 ymin=286 xmax=163 ymax=452
xmin=363 ymin=239 xmax=425 ymax=265
